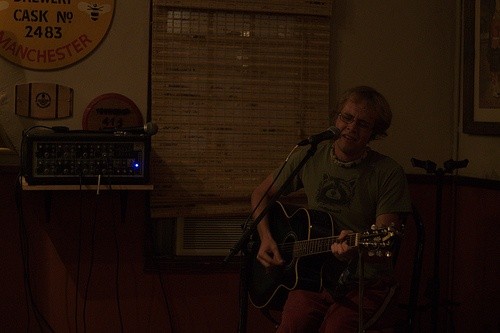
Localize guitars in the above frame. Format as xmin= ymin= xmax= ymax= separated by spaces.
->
xmin=241 ymin=198 xmax=403 ymax=309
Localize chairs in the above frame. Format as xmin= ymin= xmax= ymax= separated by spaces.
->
xmin=239 ymin=204 xmax=426 ymax=333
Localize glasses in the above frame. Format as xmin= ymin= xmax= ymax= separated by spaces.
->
xmin=337 ymin=111 xmax=373 ymax=129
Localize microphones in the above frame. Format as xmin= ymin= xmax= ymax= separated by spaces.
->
xmin=297 ymin=126 xmax=341 ymax=146
xmin=112 ymin=122 xmax=158 ymax=135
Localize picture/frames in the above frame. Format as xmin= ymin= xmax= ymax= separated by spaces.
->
xmin=463 ymin=0 xmax=500 ymax=136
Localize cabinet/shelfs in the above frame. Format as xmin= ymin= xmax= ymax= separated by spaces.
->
xmin=14 ymin=183 xmax=154 ymax=224
